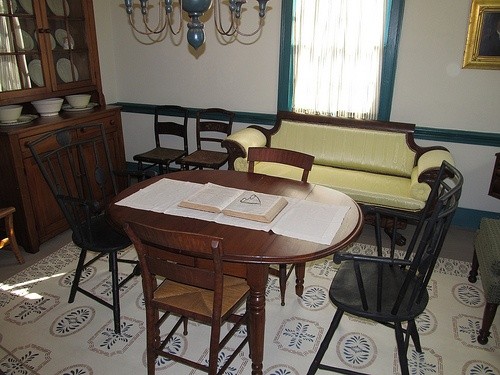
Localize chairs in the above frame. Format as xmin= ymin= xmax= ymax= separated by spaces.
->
xmin=177 ymin=106 xmax=236 ymax=170
xmin=306 ymin=159 xmax=464 ymax=375
xmin=133 ymin=104 xmax=190 ymax=172
xmin=0 ymin=207 xmax=26 ymax=265
xmin=467 ymin=216 xmax=499 ymax=342
xmin=121 ymin=219 xmax=251 ymax=375
xmin=24 ymin=121 xmax=141 ymax=334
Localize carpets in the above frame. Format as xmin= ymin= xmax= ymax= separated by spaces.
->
xmin=0 ymin=242 xmax=500 ymax=375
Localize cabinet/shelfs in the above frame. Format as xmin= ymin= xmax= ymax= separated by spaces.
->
xmin=0 ymin=0 xmax=125 ymax=254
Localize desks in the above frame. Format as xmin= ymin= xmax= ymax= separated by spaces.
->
xmin=108 ymin=170 xmax=364 ymax=375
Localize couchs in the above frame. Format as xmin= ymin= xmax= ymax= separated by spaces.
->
xmin=221 ymin=110 xmax=455 ymax=246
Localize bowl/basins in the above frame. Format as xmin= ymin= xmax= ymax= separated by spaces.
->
xmin=31 ymin=98 xmax=64 ymax=116
xmin=0 ymin=105 xmax=24 ymax=123
xmin=66 ymin=95 xmax=92 ymax=109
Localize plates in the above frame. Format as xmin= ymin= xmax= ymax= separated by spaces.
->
xmin=0 ymin=61 xmax=22 ymax=90
xmin=28 ymin=59 xmax=44 ymax=88
xmin=35 ymin=30 xmax=56 ymax=50
xmin=62 ymin=103 xmax=99 ymax=112
xmin=22 ymin=30 xmax=34 ymax=51
xmin=0 ymin=0 xmax=18 ymax=15
xmin=56 ymin=58 xmax=79 ymax=83
xmin=19 ymin=0 xmax=33 ymax=15
xmin=0 ymin=114 xmax=38 ymax=126
xmin=54 ymin=29 xmax=75 ymax=50
xmin=46 ymin=0 xmax=70 ymax=17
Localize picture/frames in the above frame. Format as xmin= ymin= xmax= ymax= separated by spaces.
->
xmin=461 ymin=0 xmax=500 ymax=70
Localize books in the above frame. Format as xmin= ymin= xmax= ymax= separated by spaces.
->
xmin=176 ymin=182 xmax=288 ymax=223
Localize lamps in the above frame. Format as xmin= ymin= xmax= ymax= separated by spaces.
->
xmin=125 ymin=0 xmax=269 ymax=52
